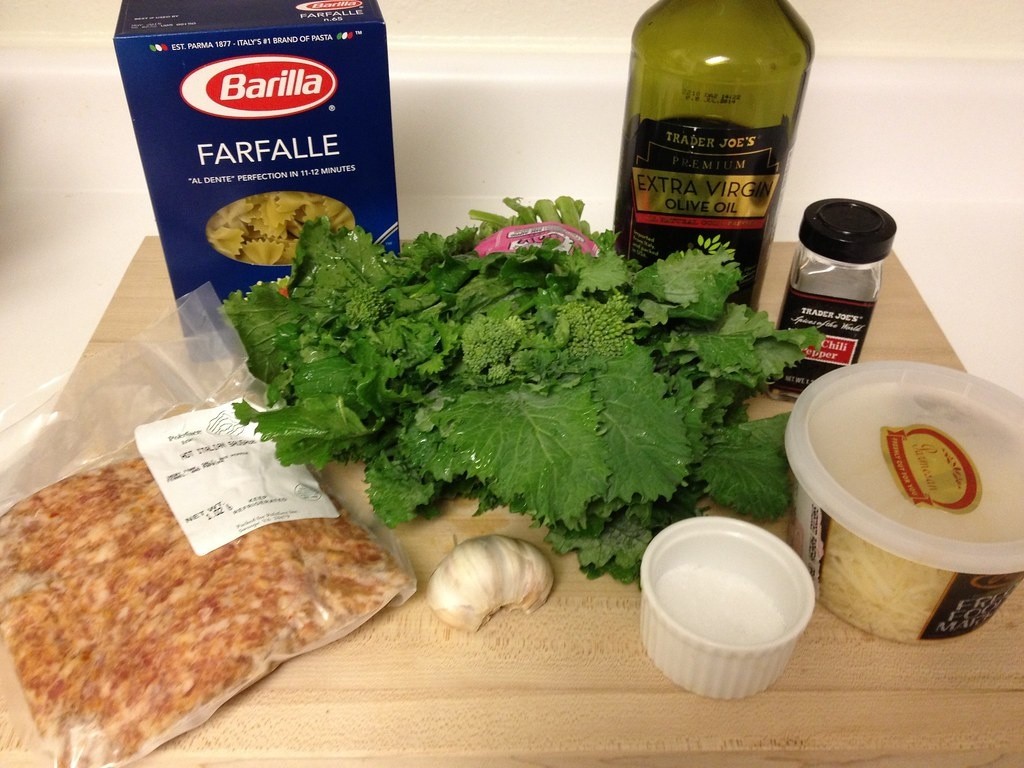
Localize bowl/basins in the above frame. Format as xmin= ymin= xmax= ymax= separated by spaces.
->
xmin=639 ymin=516 xmax=816 ymax=701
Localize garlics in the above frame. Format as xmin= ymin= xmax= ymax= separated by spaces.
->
xmin=426 ymin=534 xmax=553 ymax=634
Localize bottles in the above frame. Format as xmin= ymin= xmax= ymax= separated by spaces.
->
xmin=766 ymin=198 xmax=897 ymax=401
xmin=614 ymin=0 xmax=815 ymax=314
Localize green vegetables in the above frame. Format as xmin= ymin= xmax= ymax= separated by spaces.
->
xmin=214 ymin=197 xmax=827 ymax=583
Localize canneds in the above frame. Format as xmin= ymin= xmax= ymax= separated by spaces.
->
xmin=765 ymin=198 xmax=896 ymax=403
